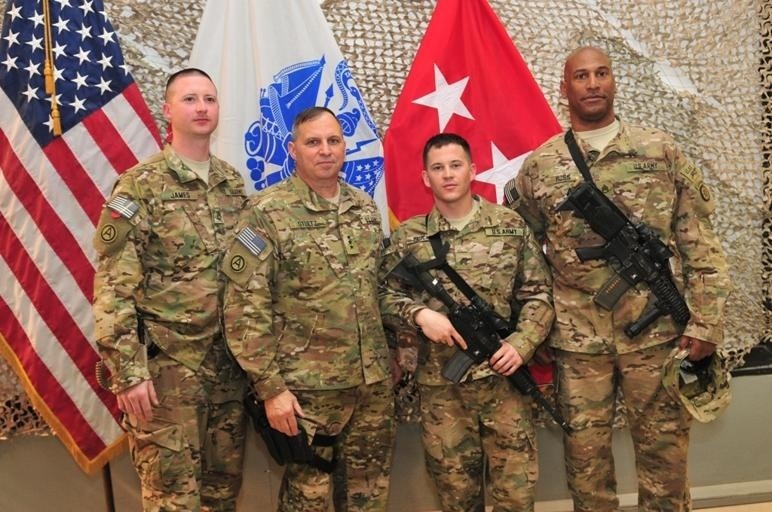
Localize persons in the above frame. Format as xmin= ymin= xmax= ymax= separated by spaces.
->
xmin=217 ymin=107 xmax=411 ymax=512
xmin=382 ymin=132 xmax=556 ymax=512
xmin=91 ymin=67 xmax=248 ymax=512
xmin=502 ymin=46 xmax=737 ymax=511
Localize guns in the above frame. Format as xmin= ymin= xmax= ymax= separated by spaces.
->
xmin=384 ymin=251 xmax=573 ymax=437
xmin=555 ymin=181 xmax=691 ymax=339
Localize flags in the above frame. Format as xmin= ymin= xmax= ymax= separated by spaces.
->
xmin=382 ymin=1 xmax=565 ymax=390
xmin=179 ymin=0 xmax=392 ymax=239
xmin=0 ymin=1 xmax=173 ymax=478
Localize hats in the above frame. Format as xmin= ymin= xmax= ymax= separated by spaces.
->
xmin=661 ymin=345 xmax=734 ymax=425
